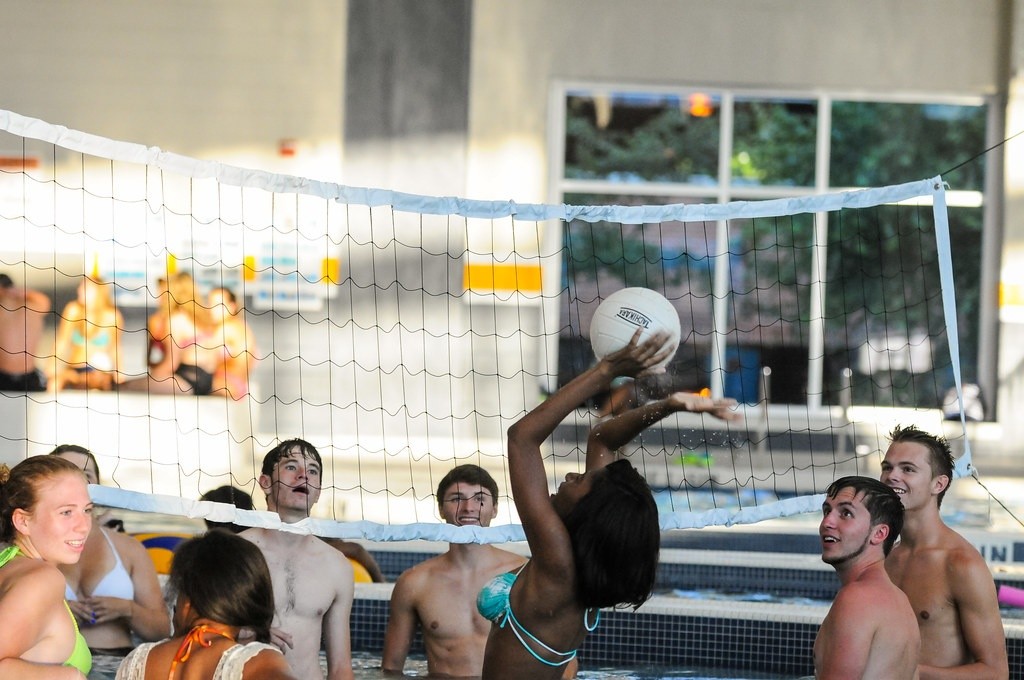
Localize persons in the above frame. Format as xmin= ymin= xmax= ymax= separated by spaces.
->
xmin=0 ymin=266 xmax=257 ymax=399
xmin=474 ymin=325 xmax=744 ymax=680
xmin=811 ymin=424 xmax=1009 ymax=680
xmin=0 ymin=439 xmax=579 ymax=679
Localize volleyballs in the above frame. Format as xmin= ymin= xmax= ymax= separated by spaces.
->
xmin=591 ymin=287 xmax=681 ymax=372
